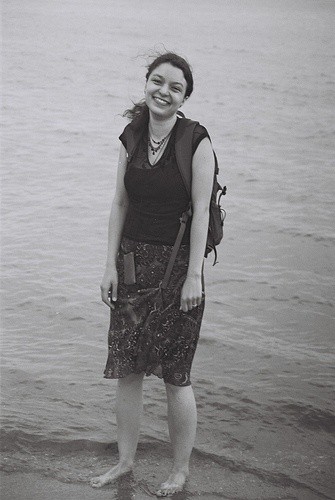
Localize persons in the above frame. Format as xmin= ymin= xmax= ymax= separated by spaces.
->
xmin=91 ymin=53 xmax=224 ymax=498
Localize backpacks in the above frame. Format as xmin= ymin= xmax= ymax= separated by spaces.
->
xmin=124 ymin=114 xmax=226 ymax=258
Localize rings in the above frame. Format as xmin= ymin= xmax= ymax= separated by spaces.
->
xmin=192 ymin=304 xmax=198 ymax=308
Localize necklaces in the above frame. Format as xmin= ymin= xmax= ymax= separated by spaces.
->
xmin=146 ymin=116 xmax=179 ymax=173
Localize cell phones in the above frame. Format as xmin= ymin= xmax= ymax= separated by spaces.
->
xmin=124 ymin=251 xmax=136 ymax=286
